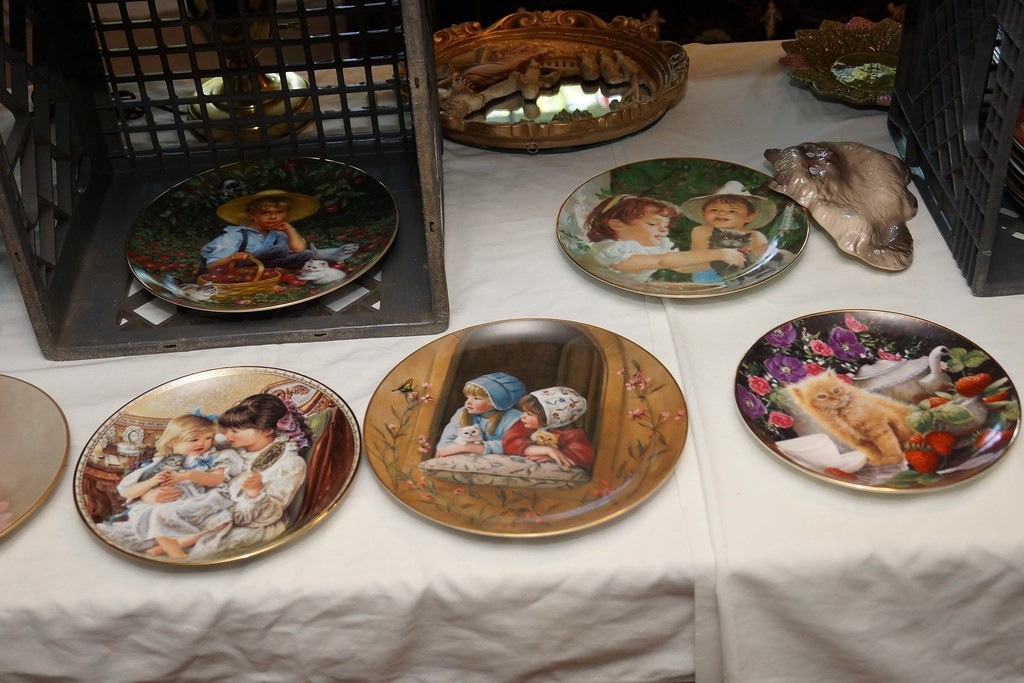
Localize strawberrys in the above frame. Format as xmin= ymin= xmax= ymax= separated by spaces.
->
xmin=903 ymin=373 xmax=1014 ymax=472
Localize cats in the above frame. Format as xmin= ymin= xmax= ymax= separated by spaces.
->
xmin=453 ymin=423 xmax=483 ymax=445
xmin=790 ymin=366 xmax=920 ymax=467
xmin=120 ymin=449 xmax=187 ymax=506
xmin=530 ymin=429 xmax=560 ymax=449
xmin=295 ymin=241 xmax=360 ymax=285
xmin=707 ymin=226 xmax=783 ymax=290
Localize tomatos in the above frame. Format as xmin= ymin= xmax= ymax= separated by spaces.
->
xmin=126 ymin=225 xmax=389 ymax=306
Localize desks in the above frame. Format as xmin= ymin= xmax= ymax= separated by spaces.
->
xmin=0 ymin=42 xmax=1024 ymax=683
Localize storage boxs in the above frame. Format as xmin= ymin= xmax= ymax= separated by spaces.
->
xmin=886 ymin=0 xmax=1024 ymax=297
xmin=0 ymin=0 xmax=449 ymax=362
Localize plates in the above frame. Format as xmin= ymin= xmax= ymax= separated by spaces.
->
xmin=0 ymin=372 xmax=70 ymax=538
xmin=553 ymin=155 xmax=812 ymax=300
xmin=363 ymin=316 xmax=690 ymax=541
xmin=733 ymin=307 xmax=1022 ymax=493
xmin=389 ymin=9 xmax=689 ymax=154
xmin=778 ymin=18 xmax=903 ymax=111
xmin=123 ymin=153 xmax=400 ymax=315
xmin=73 ymin=365 xmax=362 ymax=567
